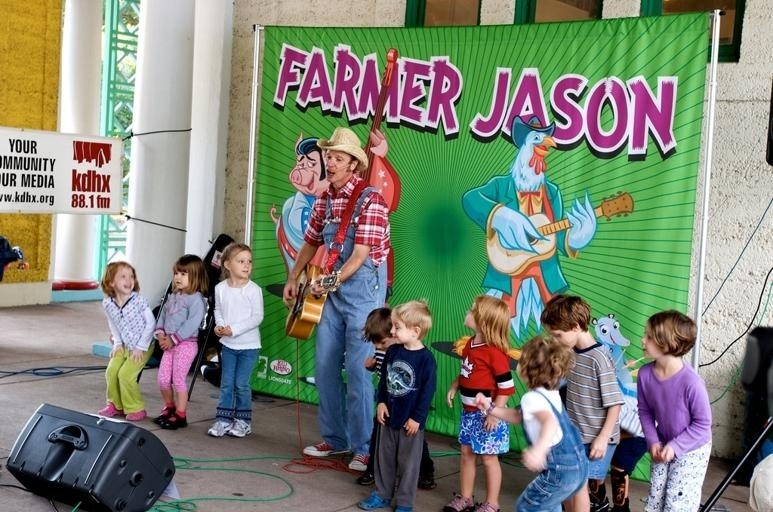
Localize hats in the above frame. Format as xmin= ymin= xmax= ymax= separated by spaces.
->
xmin=316 ymin=126 xmax=369 ymax=171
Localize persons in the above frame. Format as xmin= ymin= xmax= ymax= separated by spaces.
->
xmin=282 ymin=127 xmax=391 ymax=472
xmin=152 ymin=254 xmax=209 ymax=431
xmin=471 ymin=335 xmax=590 ymax=512
xmin=354 ymin=300 xmax=438 ymax=511
xmin=540 ymin=294 xmax=626 ymax=512
xmin=441 ymin=294 xmax=516 ymax=512
xmin=96 ymin=261 xmax=157 ymax=421
xmin=636 ymin=310 xmax=713 ymax=512
xmin=206 ymin=243 xmax=265 ymax=440
xmin=558 ymin=384 xmax=659 ymax=512
xmin=353 ymin=308 xmax=438 ymax=489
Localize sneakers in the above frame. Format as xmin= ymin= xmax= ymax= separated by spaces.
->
xmin=98 ymin=403 xmax=124 ymax=417
xmin=355 ymin=469 xmax=501 ymax=512
xmin=348 ymin=453 xmax=369 ymax=472
xmin=126 ymin=409 xmax=147 ymax=421
xmin=208 ymin=420 xmax=234 ymax=437
xmin=228 ymin=418 xmax=252 ymax=438
xmin=302 ymin=441 xmax=351 ymax=457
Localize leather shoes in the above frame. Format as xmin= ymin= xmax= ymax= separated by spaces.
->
xmin=152 ymin=408 xmax=175 ymax=424
xmin=160 ymin=414 xmax=187 ymax=430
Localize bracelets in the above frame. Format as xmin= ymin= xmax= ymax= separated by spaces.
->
xmin=483 ymin=402 xmax=496 ymax=416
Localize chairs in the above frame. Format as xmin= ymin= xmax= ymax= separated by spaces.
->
xmin=137 ymin=265 xmax=221 ymax=401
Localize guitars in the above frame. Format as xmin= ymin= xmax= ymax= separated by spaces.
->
xmin=285 ymin=263 xmax=340 ymax=339
xmin=487 ymin=191 xmax=633 ymax=276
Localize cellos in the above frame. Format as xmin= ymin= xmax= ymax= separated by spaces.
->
xmin=356 ymin=48 xmax=401 ymax=307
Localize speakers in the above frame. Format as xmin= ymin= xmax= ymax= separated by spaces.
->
xmin=5 ymin=402 xmax=176 ymax=512
xmin=203 ymin=233 xmax=236 ymax=296
